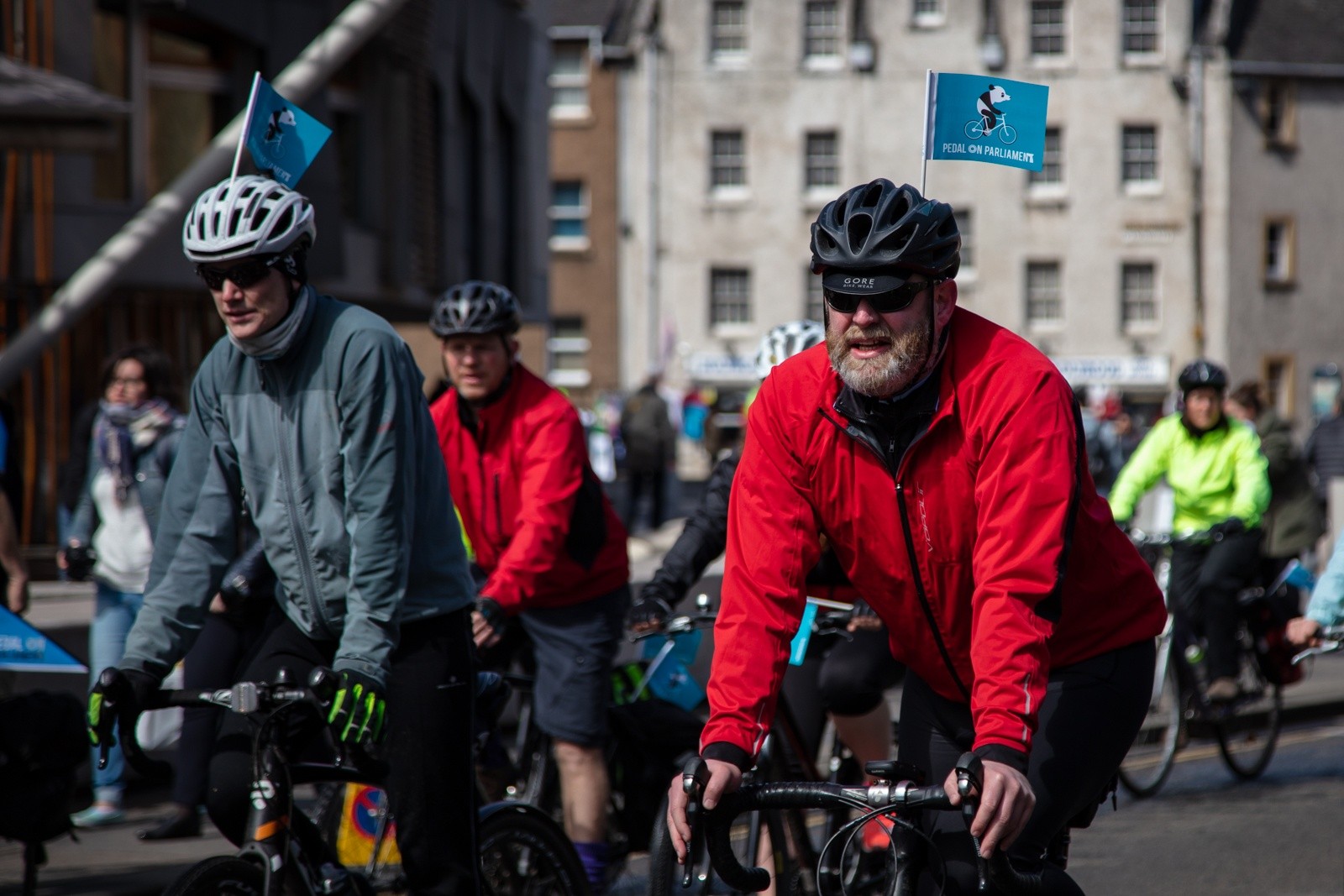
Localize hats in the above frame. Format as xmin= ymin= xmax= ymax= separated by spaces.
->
xmin=1230 ymin=382 xmax=1261 ymax=405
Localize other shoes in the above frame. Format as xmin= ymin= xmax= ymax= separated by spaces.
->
xmin=1208 ymin=679 xmax=1242 ymax=705
xmin=72 ymin=805 xmax=123 ymax=832
xmin=140 ymin=815 xmax=200 ymax=842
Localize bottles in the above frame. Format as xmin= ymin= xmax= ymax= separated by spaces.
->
xmin=66 ymin=539 xmax=89 ymax=580
xmin=321 ymin=861 xmax=363 ymax=896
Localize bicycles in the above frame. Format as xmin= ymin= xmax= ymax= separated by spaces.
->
xmin=90 ymin=665 xmax=592 ymax=896
xmin=673 ymin=755 xmax=1081 ymax=896
xmin=1117 ymin=520 xmax=1290 ymax=797
xmin=462 ymin=590 xmax=728 ymax=878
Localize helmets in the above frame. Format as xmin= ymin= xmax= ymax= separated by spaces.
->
xmin=1180 ymin=359 xmax=1226 ymax=388
xmin=752 ymin=321 xmax=827 ymax=378
xmin=182 ymin=174 xmax=317 ymax=262
xmin=812 ymin=179 xmax=961 ymax=297
xmin=433 ymin=281 xmax=523 ymax=337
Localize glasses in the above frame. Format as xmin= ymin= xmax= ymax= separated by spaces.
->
xmin=200 ymin=256 xmax=284 ymax=287
xmin=825 ymin=288 xmax=927 ymax=312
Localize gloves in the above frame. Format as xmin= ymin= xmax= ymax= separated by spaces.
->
xmin=329 ymin=670 xmax=388 ymax=744
xmin=629 ymin=586 xmax=672 ymax=624
xmin=88 ymin=670 xmax=147 ymax=749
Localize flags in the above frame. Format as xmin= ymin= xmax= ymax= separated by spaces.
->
xmin=245 ymin=72 xmax=333 ymax=189
xmin=926 ymin=71 xmax=1051 ymax=171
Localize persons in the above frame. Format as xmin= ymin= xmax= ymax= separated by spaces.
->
xmin=630 ymin=319 xmax=906 ymax=896
xmin=1108 ymin=359 xmax=1271 ymax=700
xmin=421 ymin=279 xmax=629 ymax=896
xmin=583 ymin=372 xmax=719 ymax=543
xmin=666 ymin=174 xmax=1167 ymax=896
xmin=88 ymin=176 xmax=488 ymax=896
xmin=1076 ymin=382 xmax=1344 ymax=645
xmin=0 ymin=349 xmax=285 ymax=841
